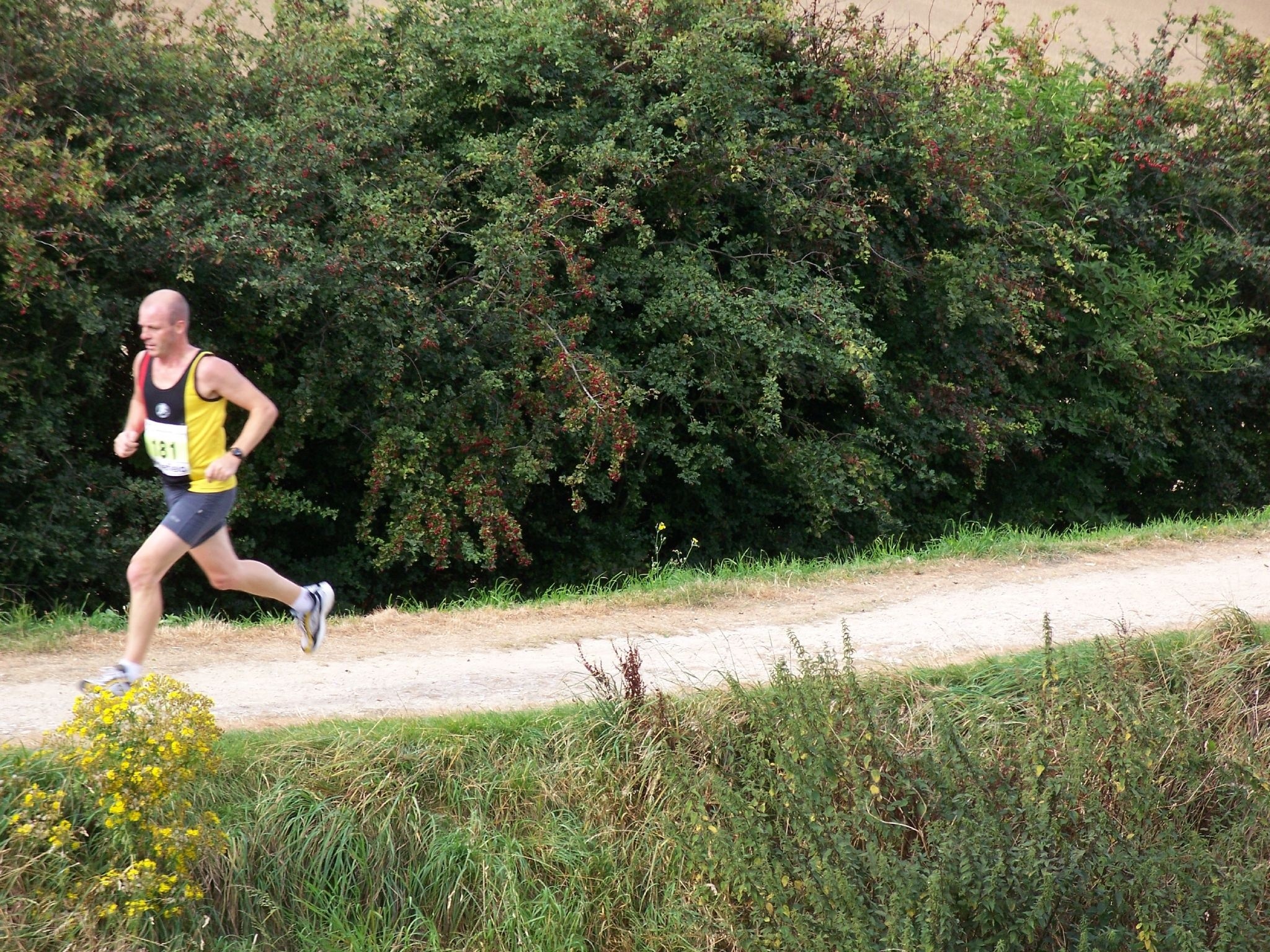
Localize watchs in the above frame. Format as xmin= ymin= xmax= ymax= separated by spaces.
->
xmin=229 ymin=447 xmax=244 ymax=460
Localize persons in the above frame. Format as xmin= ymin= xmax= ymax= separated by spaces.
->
xmin=72 ymin=289 xmax=334 ymax=705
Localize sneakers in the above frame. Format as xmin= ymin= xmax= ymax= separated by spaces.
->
xmin=289 ymin=582 xmax=334 ymax=655
xmin=80 ymin=665 xmax=129 ymax=699
